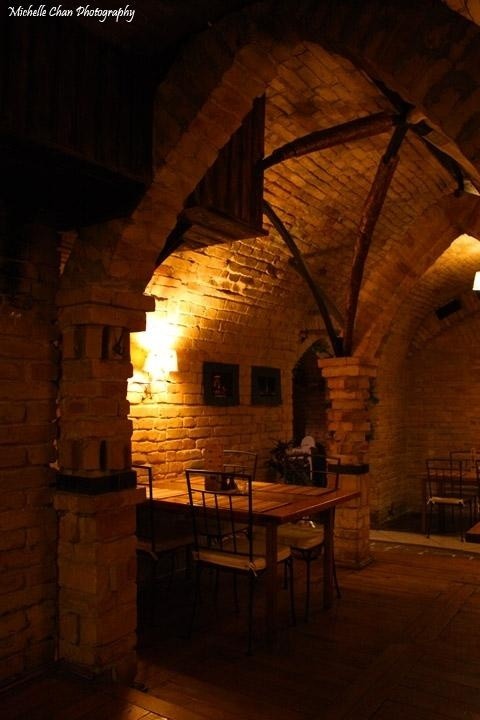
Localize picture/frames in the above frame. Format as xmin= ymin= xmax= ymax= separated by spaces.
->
xmin=251 ymin=365 xmax=282 ymax=404
xmin=203 ymin=361 xmax=240 ymax=406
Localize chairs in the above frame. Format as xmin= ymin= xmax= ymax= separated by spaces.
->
xmin=132 ymin=450 xmax=342 ymax=656
xmin=426 ymin=451 xmax=480 ymax=542
